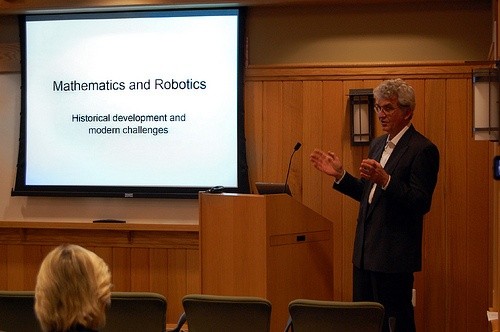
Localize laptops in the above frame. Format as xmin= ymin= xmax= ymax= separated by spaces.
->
xmin=255 ymin=182 xmax=292 ymax=197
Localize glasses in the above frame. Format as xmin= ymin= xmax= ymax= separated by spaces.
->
xmin=373 ymin=104 xmax=401 ymax=114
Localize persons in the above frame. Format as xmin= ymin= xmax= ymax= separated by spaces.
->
xmin=310 ymin=78 xmax=440 ymax=332
xmin=34 ymin=243 xmax=112 ymax=332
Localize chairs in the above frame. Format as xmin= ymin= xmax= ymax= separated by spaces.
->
xmin=0 ymin=291 xmax=396 ymax=332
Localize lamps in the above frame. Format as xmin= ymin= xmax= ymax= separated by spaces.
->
xmin=345 ymin=89 xmax=375 ymax=147
xmin=464 ymin=60 xmax=500 ymax=143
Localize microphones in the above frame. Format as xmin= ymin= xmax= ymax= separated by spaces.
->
xmin=285 ymin=142 xmax=301 ymax=194
